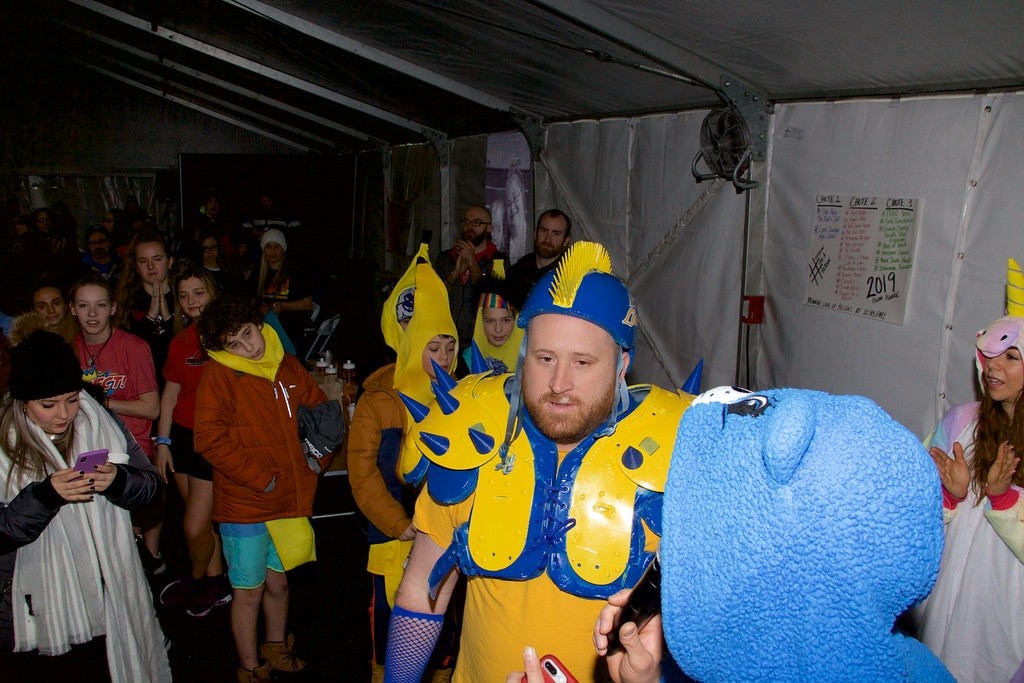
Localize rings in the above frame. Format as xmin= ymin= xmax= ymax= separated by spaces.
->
xmin=154 ymin=296 xmax=157 ymax=298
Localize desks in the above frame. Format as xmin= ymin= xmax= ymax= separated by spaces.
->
xmin=303 ymin=371 xmax=356 ymax=519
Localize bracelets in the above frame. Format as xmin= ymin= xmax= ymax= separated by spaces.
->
xmin=147 ymin=313 xmax=156 ymax=321
xmin=106 ymin=396 xmax=110 ymax=409
xmin=445 ymin=277 xmax=455 ymax=286
xmin=154 ymin=437 xmax=172 ymax=445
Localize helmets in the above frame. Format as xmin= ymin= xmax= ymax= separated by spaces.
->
xmin=517 ymin=241 xmax=637 ymax=374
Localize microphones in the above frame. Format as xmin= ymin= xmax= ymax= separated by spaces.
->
xmin=606 ymin=546 xmax=664 ymax=656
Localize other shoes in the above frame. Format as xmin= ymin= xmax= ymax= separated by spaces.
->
xmin=259 ymin=634 xmax=309 ymax=678
xmin=232 ymin=657 xmax=274 ymax=683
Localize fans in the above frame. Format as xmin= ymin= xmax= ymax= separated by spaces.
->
xmin=700 ymin=108 xmax=750 ymax=181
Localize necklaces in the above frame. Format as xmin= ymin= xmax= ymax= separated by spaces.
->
xmin=81 ymin=326 xmax=113 ymax=366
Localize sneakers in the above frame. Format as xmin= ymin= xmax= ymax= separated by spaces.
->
xmin=133 ymin=533 xmax=167 ymax=574
xmin=186 ymin=575 xmax=234 ymax=617
xmin=161 ymin=577 xmax=213 ymax=607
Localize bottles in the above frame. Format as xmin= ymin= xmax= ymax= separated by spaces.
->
xmin=315 ymin=350 xmax=357 ymax=417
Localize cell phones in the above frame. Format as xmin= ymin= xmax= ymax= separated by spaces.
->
xmin=520 ymin=654 xmax=579 ymax=683
xmin=72 ymin=449 xmax=110 ymax=481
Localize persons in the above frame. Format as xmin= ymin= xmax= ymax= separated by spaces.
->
xmin=0 ymin=191 xmax=344 ymax=682
xmin=501 ymin=209 xmax=572 ymax=313
xmin=508 ymin=256 xmax=1024 ymax=683
xmin=435 ymin=206 xmax=511 ymax=379
xmin=349 ymin=241 xmax=699 ymax=683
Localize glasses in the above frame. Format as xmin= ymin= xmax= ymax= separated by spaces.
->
xmin=87 ymin=238 xmax=107 ymax=247
xmin=101 ymin=219 xmax=114 ymax=224
xmin=202 ymin=246 xmax=218 ymax=253
xmin=460 ymin=219 xmax=489 ymax=227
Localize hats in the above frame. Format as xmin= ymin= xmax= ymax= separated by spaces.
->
xmin=12 ymin=212 xmax=32 ymax=221
xmin=6 ymin=314 xmax=83 ymax=400
xmin=973 ymin=257 xmax=1024 ymax=397
xmin=260 ymin=229 xmax=286 ymax=251
